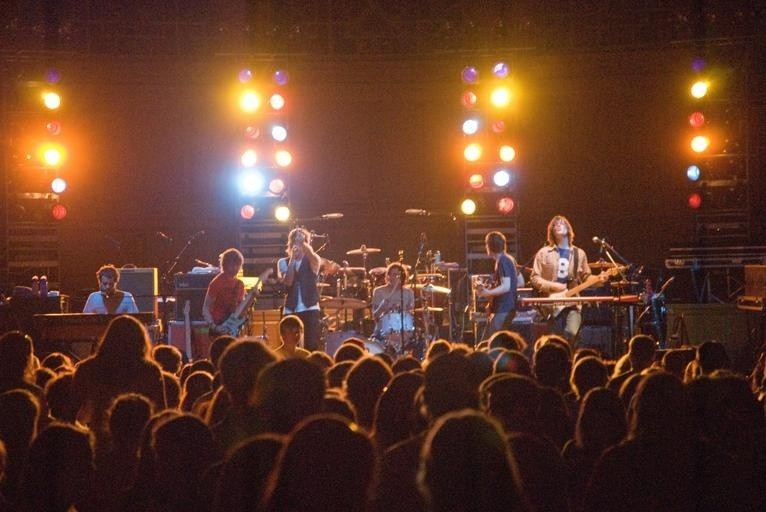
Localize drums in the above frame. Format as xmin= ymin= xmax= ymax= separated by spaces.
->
xmin=338 ymin=269 xmax=365 ymax=287
xmin=326 ymin=332 xmax=384 ymax=358
xmin=341 ymin=319 xmax=375 ymax=339
xmin=379 ymin=312 xmax=416 ymax=346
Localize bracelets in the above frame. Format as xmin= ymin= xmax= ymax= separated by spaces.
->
xmin=288 ymin=256 xmax=295 ymax=264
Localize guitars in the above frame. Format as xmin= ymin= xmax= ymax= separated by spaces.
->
xmin=538 ymin=266 xmax=630 ymax=316
xmin=209 ymin=268 xmax=274 ymax=341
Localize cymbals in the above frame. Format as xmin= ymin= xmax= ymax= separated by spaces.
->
xmin=403 ymin=284 xmax=451 ymax=293
xmin=415 ymin=274 xmax=446 ymax=279
xmin=320 ymin=298 xmax=370 ymax=308
xmin=346 ymin=248 xmax=382 ymax=254
xmin=316 ymin=283 xmax=330 ymax=287
xmin=411 ymin=307 xmax=443 ymax=312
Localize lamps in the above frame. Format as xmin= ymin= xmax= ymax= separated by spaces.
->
xmin=460 ymin=58 xmax=516 ymax=217
xmin=680 ymin=56 xmax=708 ymax=209
xmin=36 ymin=67 xmax=70 ymax=223
xmin=237 ymin=66 xmax=291 ymax=223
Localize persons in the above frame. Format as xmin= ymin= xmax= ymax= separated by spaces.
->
xmin=199 ymin=247 xmax=262 ymax=339
xmin=274 ymin=227 xmax=322 ymax=351
xmin=2 ymin=313 xmax=766 ymax=511
xmin=472 ymin=231 xmax=519 ymax=341
xmin=78 ymin=265 xmax=139 ymax=313
xmin=366 ymin=263 xmax=417 ymax=349
xmin=527 ymin=215 xmax=609 ymax=354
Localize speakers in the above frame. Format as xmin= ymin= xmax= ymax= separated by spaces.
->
xmin=116 ymin=267 xmax=158 ymax=314
xmin=175 ymin=288 xmax=214 ymax=319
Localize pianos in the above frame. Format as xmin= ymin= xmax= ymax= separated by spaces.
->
xmin=34 ymin=313 xmax=161 ymax=343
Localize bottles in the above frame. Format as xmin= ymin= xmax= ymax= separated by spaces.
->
xmin=32 ymin=274 xmax=48 ymax=299
xmin=48 ymin=290 xmax=60 ymax=297
xmin=426 ymin=249 xmax=441 ymax=264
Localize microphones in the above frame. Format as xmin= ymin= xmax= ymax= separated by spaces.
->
xmin=405 ymin=207 xmax=425 ymax=216
xmin=292 ymin=248 xmax=298 ymax=253
xmin=591 ymin=236 xmax=606 ymax=246
xmin=323 ymin=212 xmax=344 ymax=220
xmin=196 ymin=230 xmax=205 ymax=237
